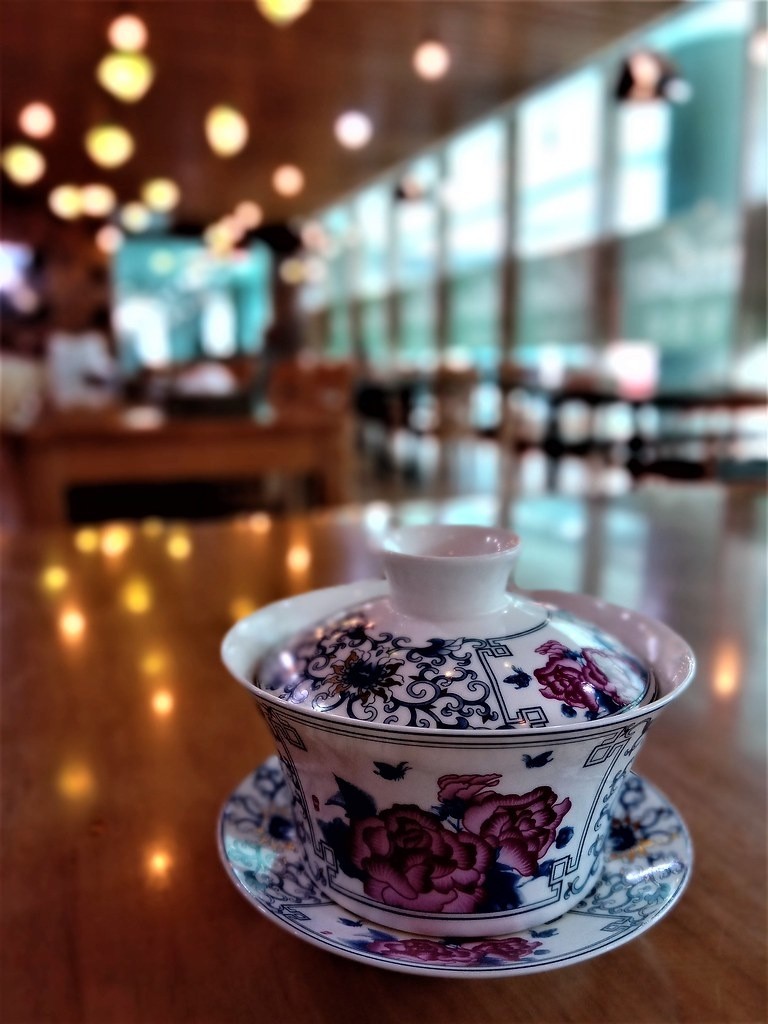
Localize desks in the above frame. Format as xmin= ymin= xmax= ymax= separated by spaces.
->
xmin=22 ymin=396 xmax=342 ymax=526
xmin=1 ymin=479 xmax=767 ymax=1024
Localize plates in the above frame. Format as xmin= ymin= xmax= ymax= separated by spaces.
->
xmin=218 ymin=753 xmax=694 ymax=980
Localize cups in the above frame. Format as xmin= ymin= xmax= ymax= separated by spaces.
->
xmin=218 ymin=521 xmax=698 ymax=937
